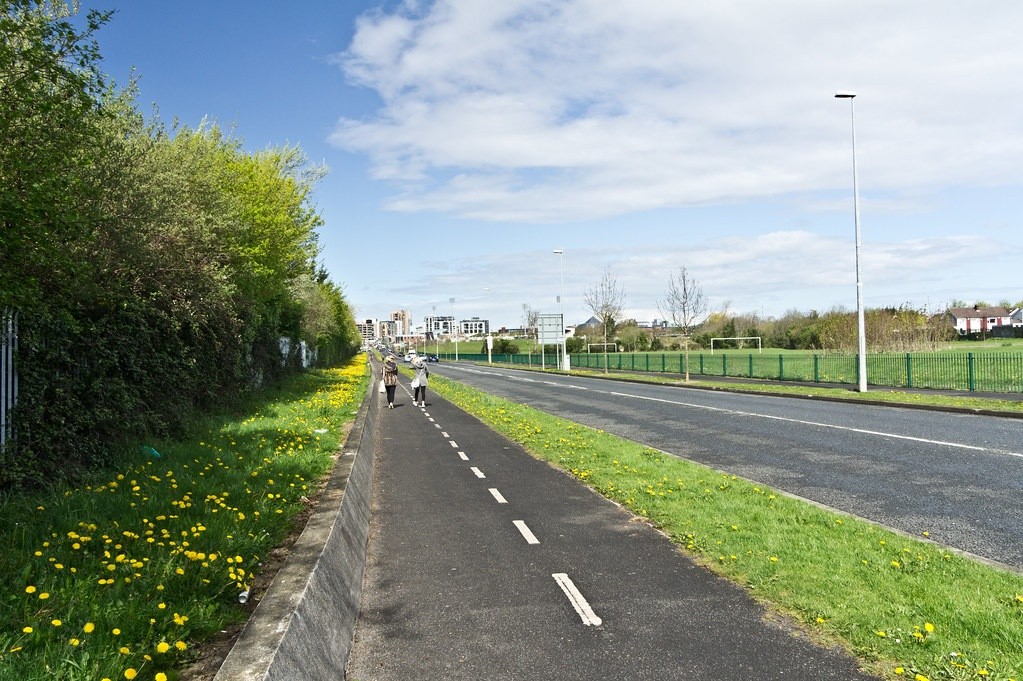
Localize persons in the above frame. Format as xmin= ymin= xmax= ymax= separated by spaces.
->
xmin=408 ymin=357 xmax=429 ymax=407
xmin=381 ymin=355 xmax=398 ymax=409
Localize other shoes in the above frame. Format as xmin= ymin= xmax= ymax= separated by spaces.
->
xmin=422 ymin=401 xmax=425 ymax=407
xmin=413 ymin=401 xmax=418 ymax=406
xmin=390 ymin=402 xmax=394 ymax=408
xmin=389 ymin=405 xmax=392 ymax=409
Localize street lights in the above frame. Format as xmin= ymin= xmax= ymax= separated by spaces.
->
xmin=834 ymin=90 xmax=868 ymax=392
xmin=553 ymin=249 xmax=567 ymax=372
xmin=483 ymin=288 xmax=491 ymax=364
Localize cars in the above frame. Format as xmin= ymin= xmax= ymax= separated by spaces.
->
xmin=398 ymin=351 xmax=439 ymax=363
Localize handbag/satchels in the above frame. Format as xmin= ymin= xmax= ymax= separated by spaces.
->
xmin=379 ymin=379 xmax=386 ymax=393
xmin=411 ymin=378 xmax=419 ymax=388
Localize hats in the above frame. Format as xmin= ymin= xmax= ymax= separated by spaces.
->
xmin=386 ymin=355 xmax=395 ymax=363
